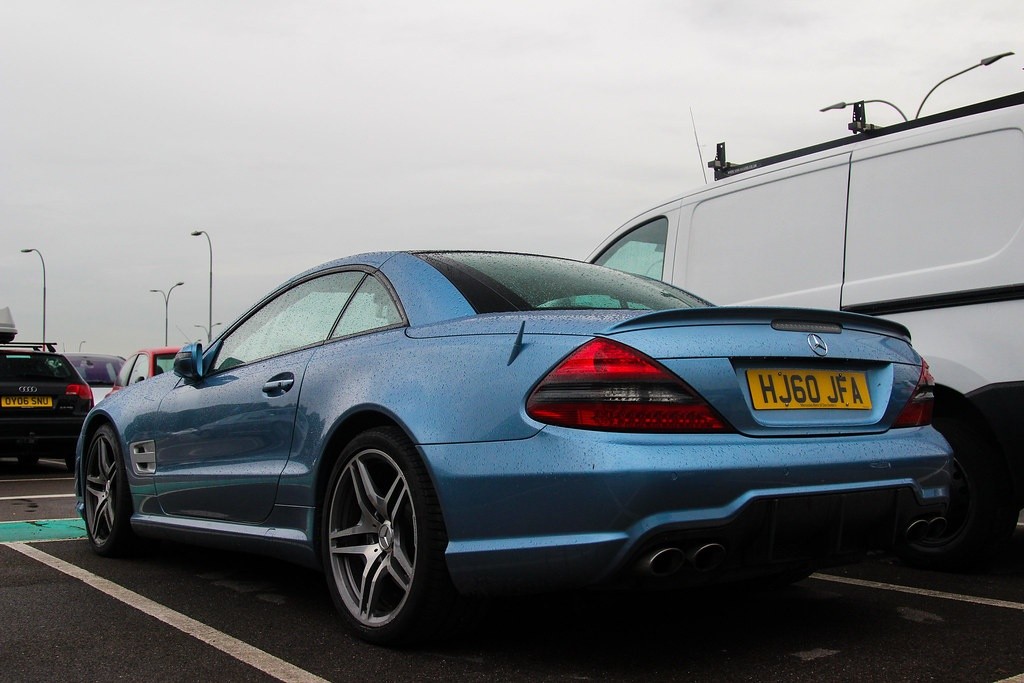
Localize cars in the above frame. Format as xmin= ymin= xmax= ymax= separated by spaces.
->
xmin=104 ymin=346 xmax=184 ymax=400
xmin=61 ymin=352 xmax=126 ymax=407
xmin=74 ymin=249 xmax=954 ymax=647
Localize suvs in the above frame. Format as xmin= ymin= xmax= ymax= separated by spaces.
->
xmin=0 ymin=307 xmax=95 ymax=473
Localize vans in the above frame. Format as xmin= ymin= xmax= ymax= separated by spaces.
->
xmin=584 ymin=96 xmax=1024 ymax=565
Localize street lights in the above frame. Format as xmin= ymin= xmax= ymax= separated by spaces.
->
xmin=817 ymin=50 xmax=1016 ymax=122
xmin=150 ymin=282 xmax=184 ymax=346
xmin=195 ymin=323 xmax=221 ymax=344
xmin=191 ymin=230 xmax=213 ymax=343
xmin=21 ymin=248 xmax=46 ymax=352
